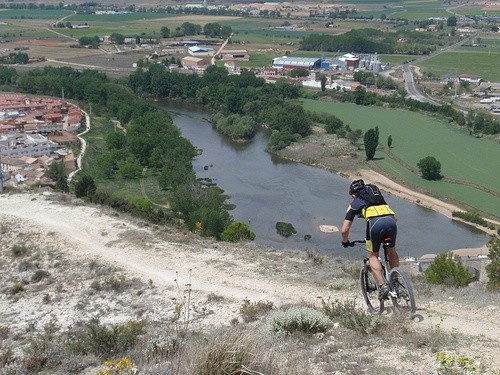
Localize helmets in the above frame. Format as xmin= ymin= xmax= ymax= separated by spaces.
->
xmin=348 ymin=178 xmax=365 ymax=193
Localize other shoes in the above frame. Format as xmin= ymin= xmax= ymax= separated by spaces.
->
xmin=377 ymin=282 xmax=390 ymax=300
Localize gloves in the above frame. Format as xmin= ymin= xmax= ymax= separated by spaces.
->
xmin=341 ymin=240 xmax=350 ymax=247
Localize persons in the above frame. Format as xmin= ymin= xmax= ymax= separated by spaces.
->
xmin=342 ymin=179 xmax=399 ymax=301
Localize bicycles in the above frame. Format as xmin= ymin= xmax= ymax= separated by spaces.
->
xmin=340 ymin=238 xmax=415 ymax=320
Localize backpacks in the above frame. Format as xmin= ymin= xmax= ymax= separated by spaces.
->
xmin=359 ymin=184 xmax=385 ymax=203
